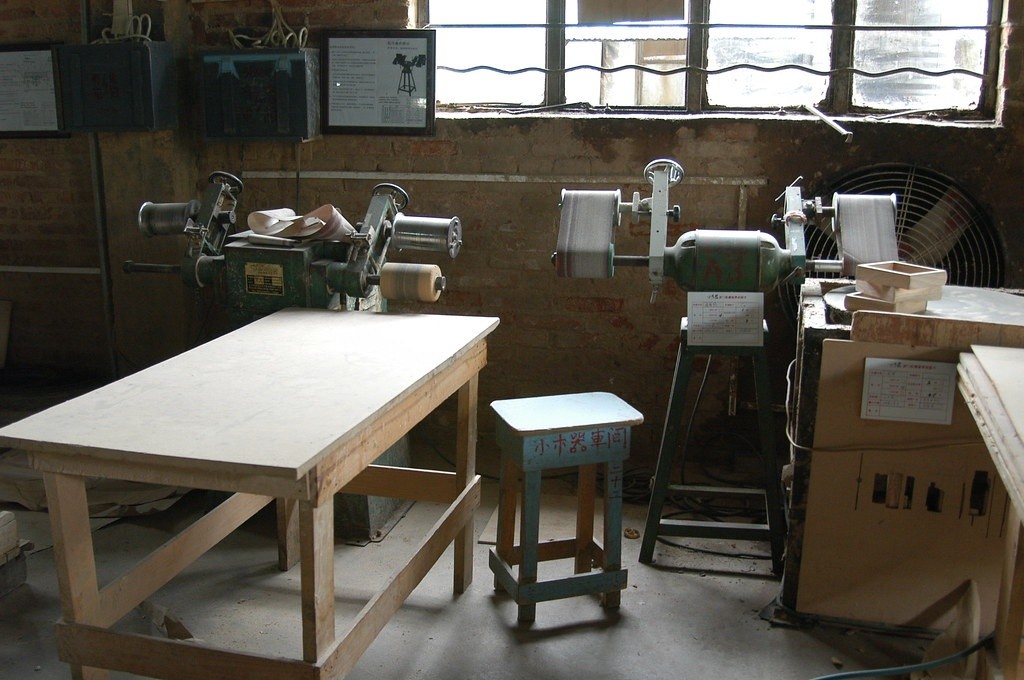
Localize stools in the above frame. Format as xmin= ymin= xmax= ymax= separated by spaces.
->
xmin=490 ymin=391 xmax=645 ymax=624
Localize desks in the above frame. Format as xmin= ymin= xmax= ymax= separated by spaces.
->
xmin=0 ymin=304 xmax=501 ymax=680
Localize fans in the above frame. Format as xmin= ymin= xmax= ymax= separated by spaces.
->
xmin=776 ymin=163 xmax=1001 ymax=330
